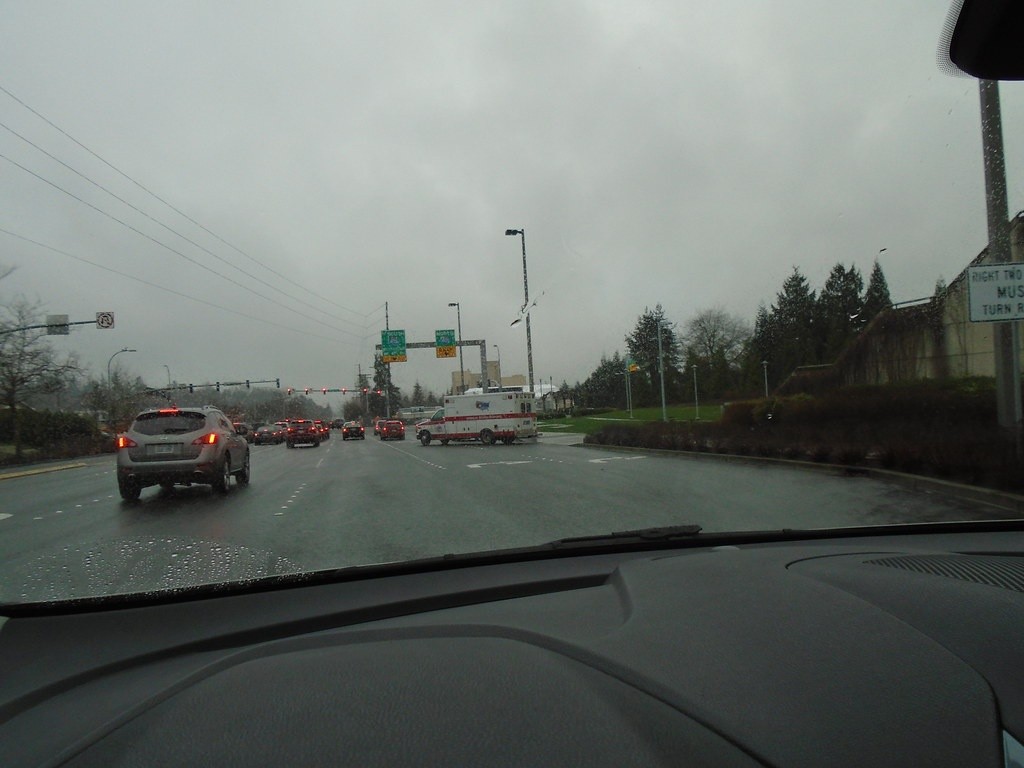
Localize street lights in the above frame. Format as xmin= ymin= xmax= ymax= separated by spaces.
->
xmin=448 ymin=302 xmax=466 ymax=394
xmin=658 ymin=319 xmax=673 ymax=422
xmin=691 ymin=364 xmax=702 ymax=420
xmin=493 ymin=344 xmax=502 ymax=392
xmin=620 ymin=369 xmax=634 ymax=419
xmin=163 ymin=365 xmax=171 ymax=385
xmin=505 ymin=228 xmax=535 ymax=398
xmin=108 ymin=349 xmax=137 ymax=389
xmin=760 ymin=361 xmax=769 ymax=396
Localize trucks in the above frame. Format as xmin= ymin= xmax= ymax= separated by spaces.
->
xmin=415 ymin=393 xmax=544 ymax=446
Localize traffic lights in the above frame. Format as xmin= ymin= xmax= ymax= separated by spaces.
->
xmin=377 ymin=390 xmax=380 ymax=394
xmin=288 ymin=388 xmax=291 ymax=392
xmin=342 ymin=388 xmax=345 ymax=392
xmin=322 ymin=388 xmax=326 ymax=391
xmin=363 ymin=388 xmax=367 ymax=392
xmin=305 ymin=389 xmax=308 ymax=391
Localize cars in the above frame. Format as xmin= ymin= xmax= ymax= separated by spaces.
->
xmin=374 ymin=420 xmax=387 ymax=435
xmin=253 ymin=418 xmax=345 ymax=447
xmin=341 ymin=420 xmax=365 ymax=440
xmin=116 ymin=406 xmax=254 ymax=503
xmin=380 ymin=420 xmax=405 ymax=440
xmin=232 ymin=422 xmax=256 ymax=443
